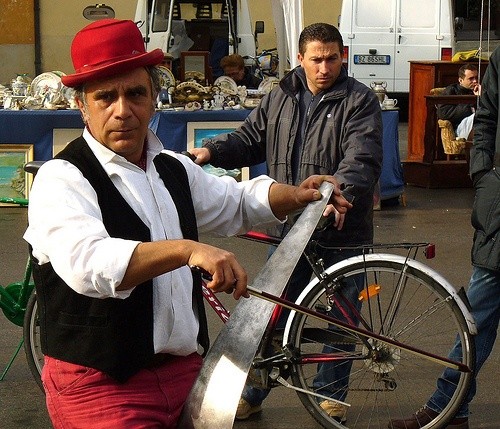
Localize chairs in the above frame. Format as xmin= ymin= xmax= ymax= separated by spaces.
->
xmin=429 ymin=87 xmax=473 ymax=162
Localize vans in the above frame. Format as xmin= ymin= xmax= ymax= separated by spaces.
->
xmin=133 ymin=0 xmax=264 ymax=86
xmin=337 ymin=0 xmax=464 ymax=93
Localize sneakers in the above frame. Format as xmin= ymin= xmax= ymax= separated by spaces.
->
xmin=319 ymin=398 xmax=347 ymax=422
xmin=388 ymin=403 xmax=469 ymax=429
xmin=235 ymin=396 xmax=262 ymax=420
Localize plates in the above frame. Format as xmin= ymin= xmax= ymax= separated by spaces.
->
xmin=154 ymin=66 xmax=175 ymax=94
xmin=175 ymin=81 xmax=207 ymax=100
xmin=214 ymin=76 xmax=238 ymax=95
xmin=29 ymin=70 xmax=67 ymax=101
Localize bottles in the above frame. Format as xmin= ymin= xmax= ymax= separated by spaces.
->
xmin=369 ymin=81 xmax=387 ymax=102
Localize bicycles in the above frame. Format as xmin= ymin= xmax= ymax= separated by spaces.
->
xmin=240 ymin=48 xmax=290 ymax=89
xmin=0 ymin=151 xmax=480 ymax=429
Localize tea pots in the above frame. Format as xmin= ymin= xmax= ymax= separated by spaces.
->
xmin=379 ymin=98 xmax=398 ymax=110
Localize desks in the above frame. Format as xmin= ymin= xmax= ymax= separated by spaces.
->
xmin=407 ymin=60 xmax=490 ymax=162
xmin=0 ymin=108 xmax=409 ymax=211
xmin=424 ymin=94 xmax=478 ymax=188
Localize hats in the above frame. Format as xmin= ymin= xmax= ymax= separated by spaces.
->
xmin=61 ymin=18 xmax=167 ymax=89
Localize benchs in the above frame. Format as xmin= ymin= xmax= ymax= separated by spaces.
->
xmin=164 ymin=3 xmax=234 ymax=73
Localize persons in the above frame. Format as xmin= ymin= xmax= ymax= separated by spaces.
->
xmin=438 ymin=63 xmax=481 ymax=175
xmin=220 ymin=54 xmax=263 ymax=90
xmin=187 ymin=22 xmax=385 ymax=424
xmin=22 ymin=18 xmax=354 ymax=429
xmin=388 ymin=47 xmax=500 ymax=429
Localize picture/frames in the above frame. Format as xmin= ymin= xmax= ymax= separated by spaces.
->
xmin=0 ymin=143 xmax=34 ymax=206
xmin=186 ymin=121 xmax=250 ymax=182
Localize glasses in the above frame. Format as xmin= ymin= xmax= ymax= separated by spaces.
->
xmin=224 ymin=69 xmax=240 ymax=79
